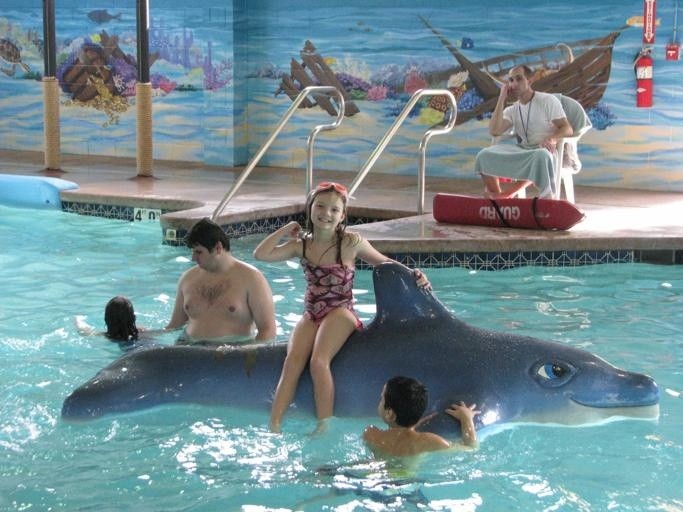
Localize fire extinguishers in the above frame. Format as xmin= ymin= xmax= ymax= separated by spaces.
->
xmin=633 ymin=47 xmax=655 ymax=107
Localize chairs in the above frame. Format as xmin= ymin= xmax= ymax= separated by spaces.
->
xmin=484 ymin=93 xmax=587 ymax=205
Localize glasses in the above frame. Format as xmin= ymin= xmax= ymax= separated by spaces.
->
xmin=311 ymin=182 xmax=348 ymax=204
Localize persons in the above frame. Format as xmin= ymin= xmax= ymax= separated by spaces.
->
xmin=131 ymin=216 xmax=279 ymax=347
xmin=250 ymin=181 xmax=434 ymax=442
xmin=476 ymin=64 xmax=574 ymax=201
xmin=288 ymin=375 xmax=485 ymax=512
xmin=76 ymin=296 xmax=145 ymax=341
